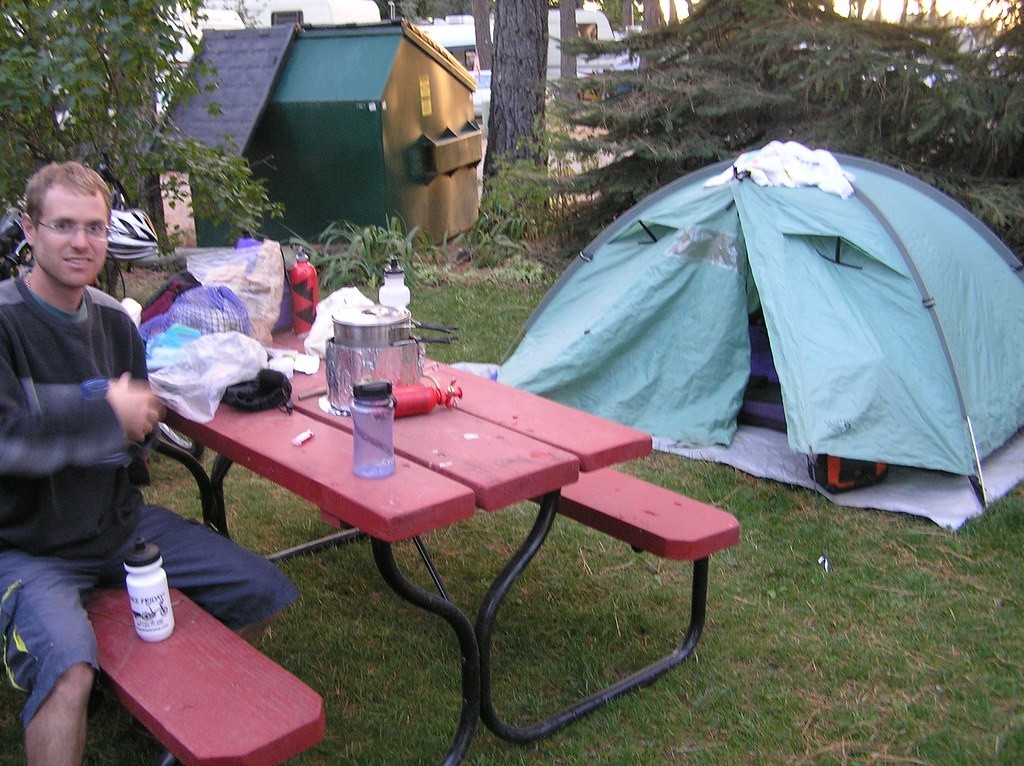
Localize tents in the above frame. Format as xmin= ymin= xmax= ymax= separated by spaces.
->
xmin=497 ymin=147 xmax=1023 ymax=534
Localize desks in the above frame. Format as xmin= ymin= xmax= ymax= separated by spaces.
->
xmin=150 ymin=329 xmax=653 ymax=766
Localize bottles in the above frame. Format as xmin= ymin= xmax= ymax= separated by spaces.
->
xmin=123 ymin=535 xmax=174 ymax=644
xmin=79 ymin=374 xmax=130 ymax=467
xmin=289 ymin=245 xmax=318 ymax=334
xmin=378 ymin=259 xmax=410 ymax=308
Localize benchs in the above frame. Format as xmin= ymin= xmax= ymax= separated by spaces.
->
xmin=79 ymin=579 xmax=327 ymax=766
xmin=528 ymin=462 xmax=740 ymax=652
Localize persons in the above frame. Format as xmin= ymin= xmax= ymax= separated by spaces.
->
xmin=0 ymin=161 xmax=301 ymax=766
xmin=737 ymin=303 xmax=787 ymax=435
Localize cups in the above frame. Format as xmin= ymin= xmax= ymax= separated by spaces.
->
xmin=349 ymin=381 xmax=397 ymax=477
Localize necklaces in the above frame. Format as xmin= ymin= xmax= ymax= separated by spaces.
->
xmin=25 ymin=271 xmax=31 ymax=288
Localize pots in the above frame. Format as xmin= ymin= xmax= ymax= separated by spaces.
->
xmin=331 ymin=304 xmax=457 ymax=348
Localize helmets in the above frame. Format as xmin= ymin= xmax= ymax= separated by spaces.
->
xmin=106 ymin=209 xmax=159 ymax=260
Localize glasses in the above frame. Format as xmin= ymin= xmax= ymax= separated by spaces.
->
xmin=29 ymin=215 xmax=110 ymax=236
